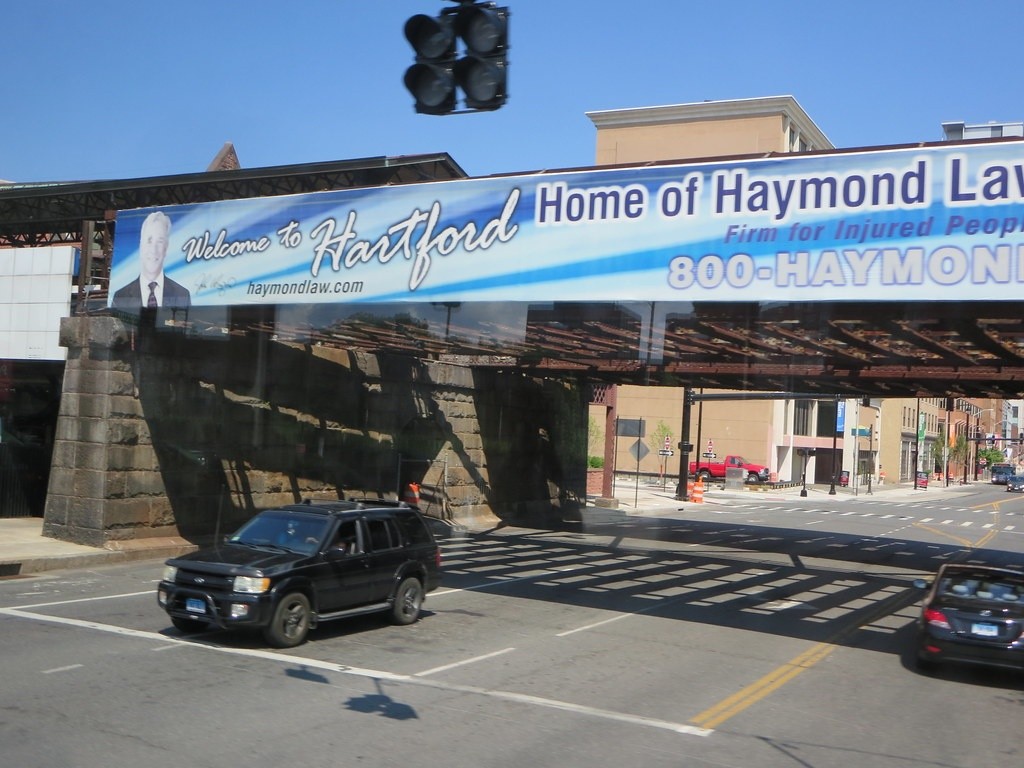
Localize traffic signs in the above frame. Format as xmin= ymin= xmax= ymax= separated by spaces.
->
xmin=703 ymin=453 xmax=716 ymax=458
xmin=659 ymin=450 xmax=673 ymax=456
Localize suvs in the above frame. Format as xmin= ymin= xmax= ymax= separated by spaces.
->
xmin=157 ymin=497 xmax=441 ymax=648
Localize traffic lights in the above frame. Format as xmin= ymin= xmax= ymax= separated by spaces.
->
xmin=452 ymin=4 xmax=508 ymax=111
xmin=809 ymin=450 xmax=816 ymax=456
xmin=797 ymin=449 xmax=804 ymax=456
xmin=403 ymin=14 xmax=456 ymax=115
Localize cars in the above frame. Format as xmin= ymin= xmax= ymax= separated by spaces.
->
xmin=1007 ymin=475 xmax=1024 ymax=493
xmin=914 ymin=563 xmax=1024 ymax=676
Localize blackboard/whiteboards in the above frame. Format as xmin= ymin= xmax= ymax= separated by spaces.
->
xmin=916 ymin=470 xmax=929 ymax=487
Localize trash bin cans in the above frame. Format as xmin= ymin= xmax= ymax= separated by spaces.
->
xmin=839 ymin=470 xmax=850 ymax=487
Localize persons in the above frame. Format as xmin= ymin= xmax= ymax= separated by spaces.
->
xmin=277 ymin=519 xmax=346 ymax=553
xmin=110 ymin=212 xmax=192 ymax=335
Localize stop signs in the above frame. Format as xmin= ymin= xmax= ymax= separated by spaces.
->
xmin=707 ymin=441 xmax=714 ymax=453
xmin=665 ymin=437 xmax=670 ymax=450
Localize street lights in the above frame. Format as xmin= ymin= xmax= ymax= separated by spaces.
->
xmin=964 ymin=407 xmax=971 ymax=483
xmin=994 ymin=420 xmax=1012 ymax=435
xmin=970 ymin=409 xmax=994 ymax=481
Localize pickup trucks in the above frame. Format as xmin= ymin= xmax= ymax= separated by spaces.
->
xmin=690 ymin=455 xmax=770 ymax=482
